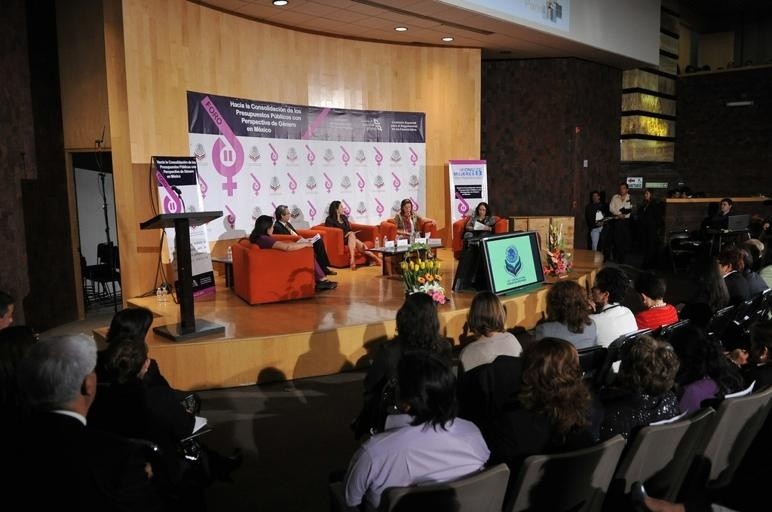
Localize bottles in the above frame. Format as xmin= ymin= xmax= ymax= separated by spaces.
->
xmin=227 ymin=246 xmax=232 ymax=260
xmin=157 ymin=288 xmax=161 ymax=302
xmin=383 ymin=235 xmax=388 ymax=248
xmin=395 ymin=234 xmax=399 ymax=241
xmin=375 ymin=237 xmax=379 ymax=249
xmin=163 ymin=287 xmax=168 ymax=301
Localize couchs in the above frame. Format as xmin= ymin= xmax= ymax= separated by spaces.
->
xmin=232 ymin=238 xmax=313 ymax=306
xmin=311 ymin=224 xmax=378 ymax=268
xmin=454 ymin=217 xmax=510 ymax=259
xmin=270 ymin=230 xmax=327 ymax=256
xmin=381 ymin=219 xmax=437 ymax=258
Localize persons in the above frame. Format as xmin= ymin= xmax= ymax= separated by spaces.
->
xmin=326 ymin=202 xmax=384 ymax=270
xmin=705 ymin=199 xmax=739 ymax=245
xmin=609 ymin=183 xmax=633 ymax=219
xmin=393 ymin=198 xmax=422 ymax=240
xmin=1 ymin=217 xmax=771 ymax=512
xmin=273 ymin=205 xmax=337 ymax=275
xmin=464 ymin=202 xmax=496 ymax=251
xmin=249 ymin=216 xmax=338 ymax=291
xmin=666 ymin=175 xmax=697 ymax=197
xmin=638 ymin=190 xmax=657 ymax=218
xmin=585 ymin=191 xmax=608 ymax=251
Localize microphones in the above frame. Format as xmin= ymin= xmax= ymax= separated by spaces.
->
xmin=172 ymin=185 xmax=182 ymax=194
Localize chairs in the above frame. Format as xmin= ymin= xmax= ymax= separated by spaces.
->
xmin=456 ymin=364 xmax=495 ymax=422
xmin=575 ymin=216 xmax=770 ymax=393
xmin=80 ymin=240 xmax=122 ymax=313
xmin=512 ymin=435 xmax=628 ymax=510
xmin=493 ymin=355 xmax=530 ymax=413
xmin=606 ymin=406 xmax=715 ymax=510
xmin=382 ymin=463 xmax=511 ymax=510
xmin=692 ymin=394 xmax=770 ymax=492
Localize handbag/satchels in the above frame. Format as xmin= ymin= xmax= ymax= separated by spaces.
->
xmin=171 ymin=389 xmax=201 ymax=414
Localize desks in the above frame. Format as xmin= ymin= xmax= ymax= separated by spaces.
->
xmin=212 ymin=256 xmax=232 ymax=287
xmin=364 ymin=243 xmax=444 ymax=276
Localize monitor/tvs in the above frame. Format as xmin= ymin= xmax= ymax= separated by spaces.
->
xmin=481 ymin=230 xmax=546 ymax=296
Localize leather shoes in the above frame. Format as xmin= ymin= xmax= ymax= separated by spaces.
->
xmin=324 ymin=268 xmax=337 ymax=275
xmin=316 ymin=280 xmax=337 ymax=289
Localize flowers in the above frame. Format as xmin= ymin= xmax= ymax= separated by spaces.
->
xmin=542 ymin=222 xmax=575 ymax=278
xmin=399 ymin=244 xmax=446 ymax=306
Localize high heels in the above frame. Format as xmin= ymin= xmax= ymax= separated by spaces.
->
xmin=218 ymin=447 xmax=245 ymax=485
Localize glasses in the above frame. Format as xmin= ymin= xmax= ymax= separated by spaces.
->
xmin=285 ymin=211 xmax=292 ymax=215
